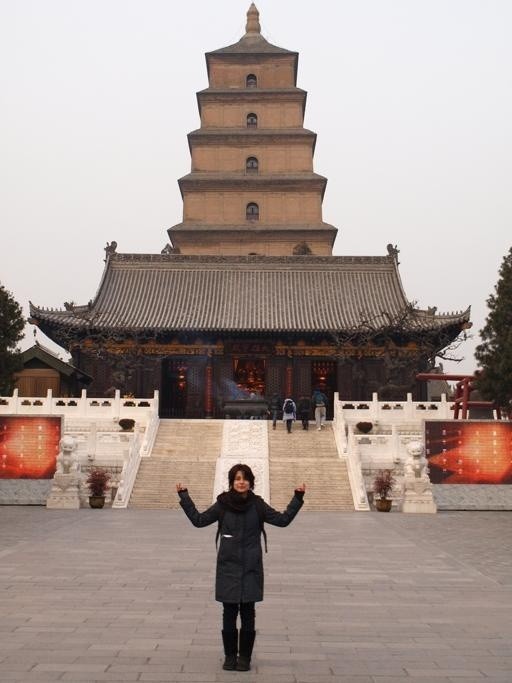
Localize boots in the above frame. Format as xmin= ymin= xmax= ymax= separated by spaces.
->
xmin=221 ymin=629 xmax=256 ymax=671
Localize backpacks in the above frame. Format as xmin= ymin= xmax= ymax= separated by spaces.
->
xmin=302 ymin=399 xmax=309 ymax=409
xmin=284 ymin=400 xmax=293 ymax=413
xmin=315 ymin=393 xmax=323 ymax=404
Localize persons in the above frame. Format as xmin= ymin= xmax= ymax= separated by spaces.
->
xmin=283 ymin=393 xmax=296 ymax=434
xmin=269 ymin=392 xmax=280 ymax=431
xmin=176 ymin=464 xmax=305 ymax=673
xmin=297 ymin=394 xmax=312 ymax=430
xmin=312 ymin=386 xmax=330 ymax=431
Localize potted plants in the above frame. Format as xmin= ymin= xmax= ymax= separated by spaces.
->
xmin=85 ymin=464 xmax=111 ymax=508
xmin=372 ymin=470 xmax=398 ymax=512
